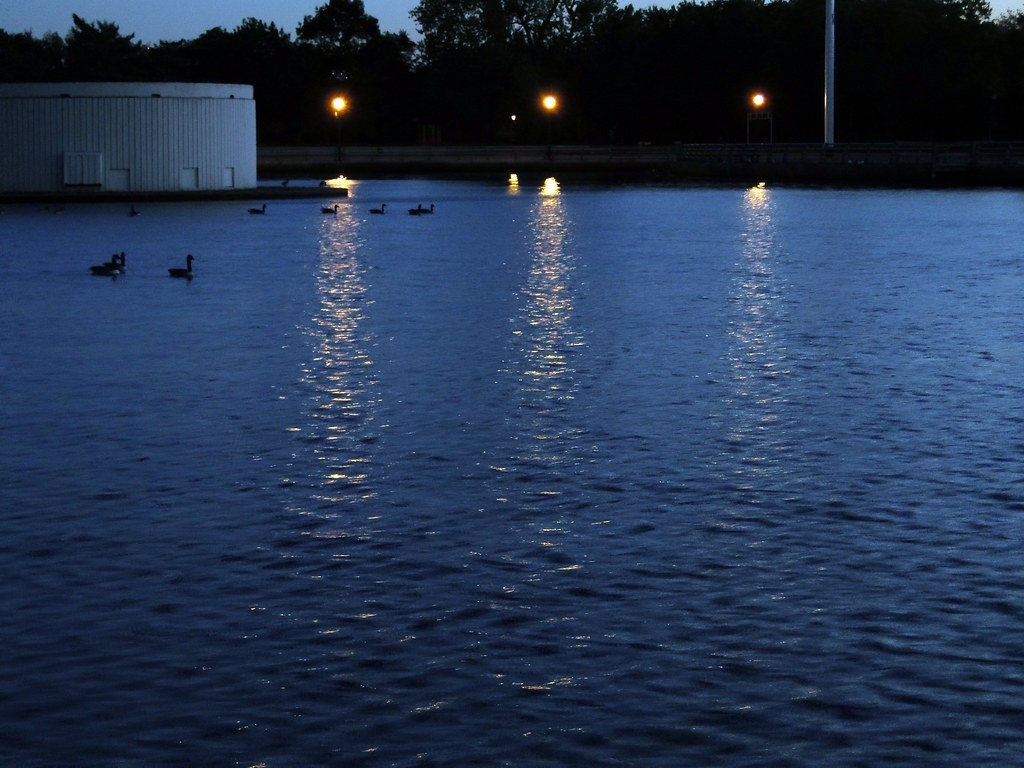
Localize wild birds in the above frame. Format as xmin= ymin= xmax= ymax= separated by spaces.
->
xmin=408 ymin=203 xmax=435 ymax=216
xmin=168 ymin=254 xmax=196 ymax=278
xmin=320 ymin=204 xmax=339 ymax=215
xmin=281 ymin=179 xmax=290 ymax=186
xmin=319 ymin=180 xmax=326 ymax=188
xmin=247 ymin=204 xmax=267 ymax=216
xmin=368 ymin=203 xmax=387 ymax=215
xmin=127 ymin=205 xmax=141 ymax=216
xmin=88 ymin=251 xmax=126 ymax=278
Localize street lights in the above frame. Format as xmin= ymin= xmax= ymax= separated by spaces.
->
xmin=542 ymin=96 xmax=558 ymax=147
xmin=331 ymin=96 xmax=347 ymax=161
